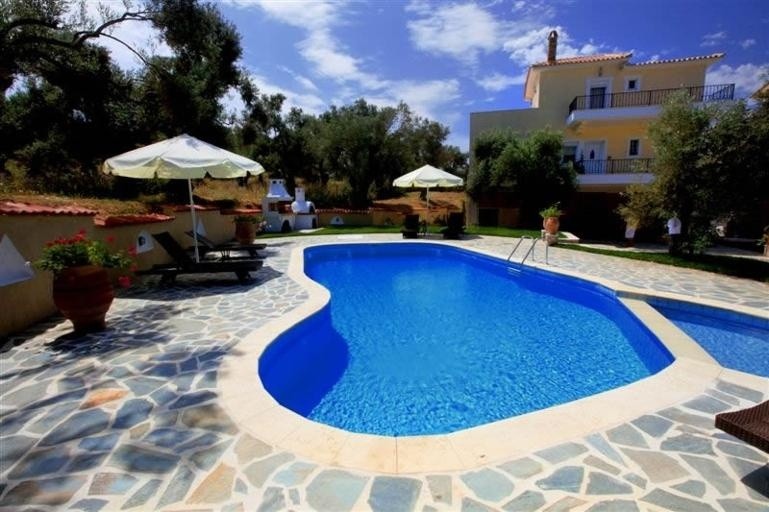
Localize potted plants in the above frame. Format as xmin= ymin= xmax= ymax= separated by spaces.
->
xmin=539 ymin=201 xmax=566 ymax=234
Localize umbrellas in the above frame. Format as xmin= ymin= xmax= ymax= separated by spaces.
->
xmin=100 ymin=129 xmax=266 ymax=263
xmin=392 ymin=161 xmax=464 ymax=235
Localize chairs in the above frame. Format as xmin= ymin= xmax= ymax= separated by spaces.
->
xmin=400 ymin=215 xmax=420 ymax=238
xmin=441 ymin=212 xmax=465 ymax=239
xmin=135 ymin=230 xmax=267 ymax=288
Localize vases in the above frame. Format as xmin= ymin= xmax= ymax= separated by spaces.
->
xmin=235 ymin=222 xmax=256 ymax=244
xmin=53 ymin=264 xmax=115 ymax=334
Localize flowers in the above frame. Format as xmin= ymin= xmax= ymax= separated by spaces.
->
xmin=27 ymin=226 xmax=139 ymax=291
xmin=232 ymin=214 xmax=264 ymax=224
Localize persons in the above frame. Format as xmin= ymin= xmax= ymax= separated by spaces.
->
xmin=667 ymin=216 xmax=682 ymax=257
